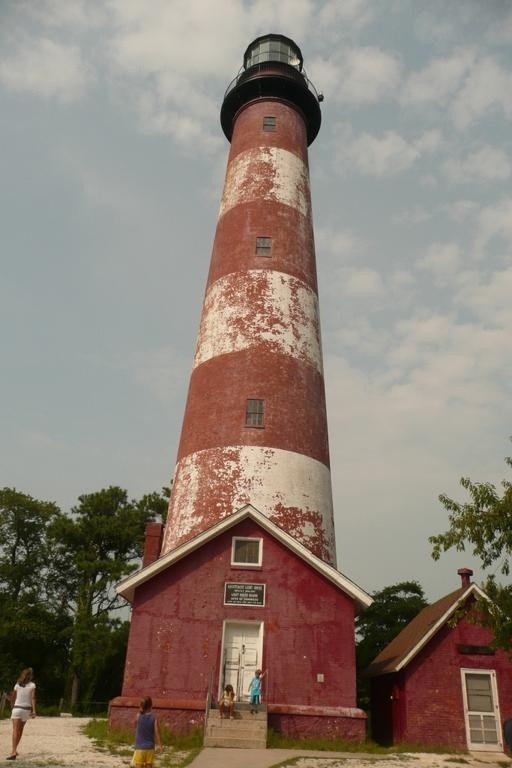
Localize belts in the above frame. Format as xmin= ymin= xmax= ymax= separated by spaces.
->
xmin=13 ymin=705 xmax=32 ymax=710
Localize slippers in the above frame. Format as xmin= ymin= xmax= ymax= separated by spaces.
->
xmin=6 ymin=752 xmax=19 ymax=760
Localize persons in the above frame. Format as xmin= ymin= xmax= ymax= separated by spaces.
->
xmin=6 ymin=667 xmax=37 ymax=760
xmin=248 ymin=669 xmax=269 ymax=713
xmin=218 ymin=684 xmax=235 ymax=720
xmin=133 ymin=695 xmax=165 ymax=768
